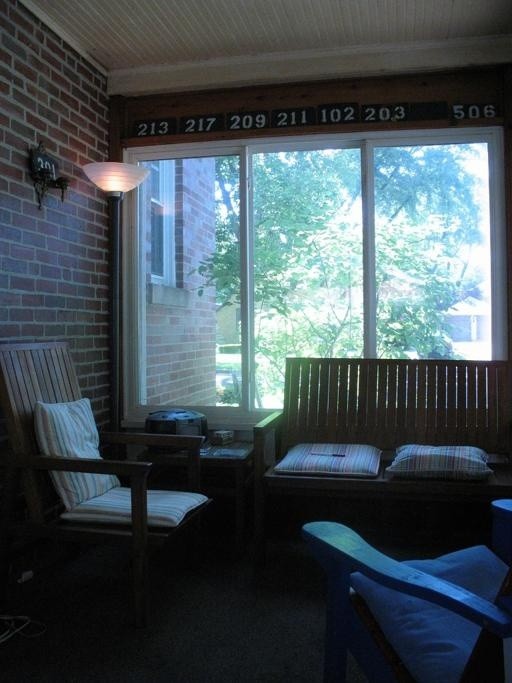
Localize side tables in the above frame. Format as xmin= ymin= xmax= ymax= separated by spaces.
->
xmin=135 ymin=439 xmax=255 ymax=554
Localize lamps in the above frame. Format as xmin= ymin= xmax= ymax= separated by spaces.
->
xmin=26 ymin=139 xmax=154 ymax=209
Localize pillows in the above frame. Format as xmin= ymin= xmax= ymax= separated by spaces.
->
xmin=58 ymin=484 xmax=209 ymax=532
xmin=32 ymin=394 xmax=123 ymax=511
xmin=346 ymin=542 xmax=512 ymax=683
xmin=272 ymin=442 xmax=382 ymax=479
xmin=387 ymin=443 xmax=495 ymax=481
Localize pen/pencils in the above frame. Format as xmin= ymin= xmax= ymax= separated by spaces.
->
xmin=310 ymin=451 xmax=345 ymax=457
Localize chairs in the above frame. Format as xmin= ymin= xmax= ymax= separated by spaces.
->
xmin=1 ymin=341 xmax=213 ymax=631
xmin=302 ymin=497 xmax=512 ymax=683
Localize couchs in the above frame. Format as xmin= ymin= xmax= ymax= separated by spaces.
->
xmin=253 ymin=354 xmax=511 ymax=543
